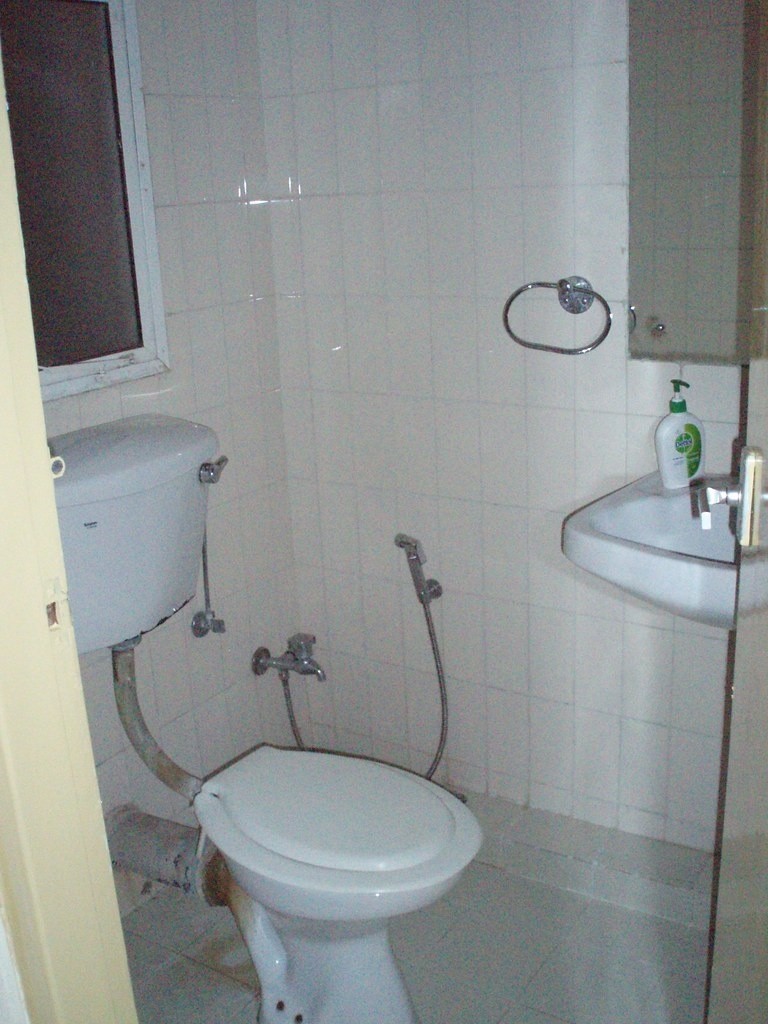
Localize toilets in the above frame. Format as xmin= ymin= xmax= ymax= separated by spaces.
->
xmin=41 ymin=413 xmax=479 ymax=1024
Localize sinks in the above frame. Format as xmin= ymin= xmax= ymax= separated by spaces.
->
xmin=563 ymin=469 xmax=768 ymax=628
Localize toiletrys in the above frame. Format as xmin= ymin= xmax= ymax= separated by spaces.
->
xmin=656 ymin=379 xmax=706 ymax=488
